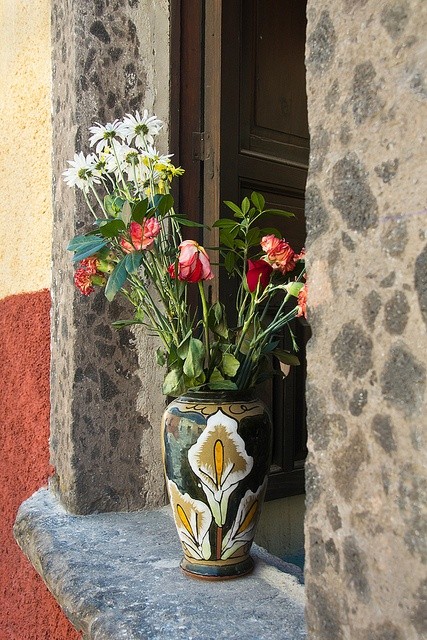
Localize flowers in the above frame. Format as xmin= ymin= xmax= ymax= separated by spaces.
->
xmin=62 ymin=111 xmax=306 ymax=398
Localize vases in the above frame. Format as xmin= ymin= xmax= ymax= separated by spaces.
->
xmin=160 ymin=390 xmax=274 ymax=581
